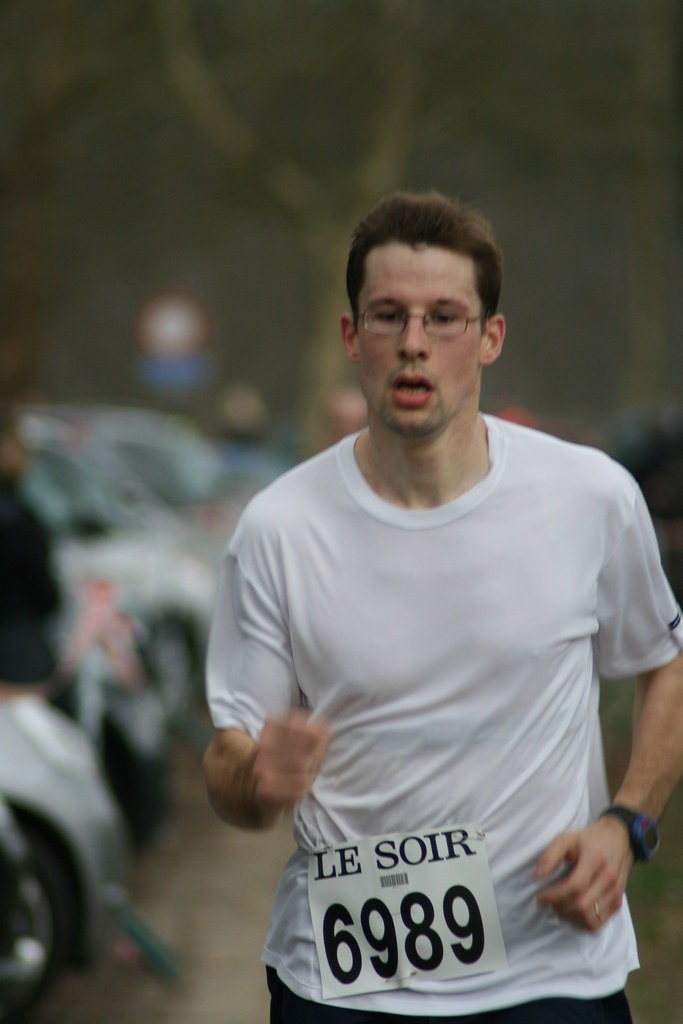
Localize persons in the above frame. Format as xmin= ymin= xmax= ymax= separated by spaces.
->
xmin=204 ymin=194 xmax=682 ymax=1023
xmin=0 ymin=432 xmax=58 ymax=686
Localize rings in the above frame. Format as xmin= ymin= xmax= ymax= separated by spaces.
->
xmin=592 ymin=902 xmax=602 ymax=923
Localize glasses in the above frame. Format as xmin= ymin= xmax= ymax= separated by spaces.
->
xmin=357 ymin=303 xmax=483 ymax=338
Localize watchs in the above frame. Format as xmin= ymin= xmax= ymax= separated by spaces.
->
xmin=596 ymin=805 xmax=660 ymax=865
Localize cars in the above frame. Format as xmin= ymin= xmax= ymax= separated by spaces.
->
xmin=1 ymin=401 xmax=297 ymax=1024
